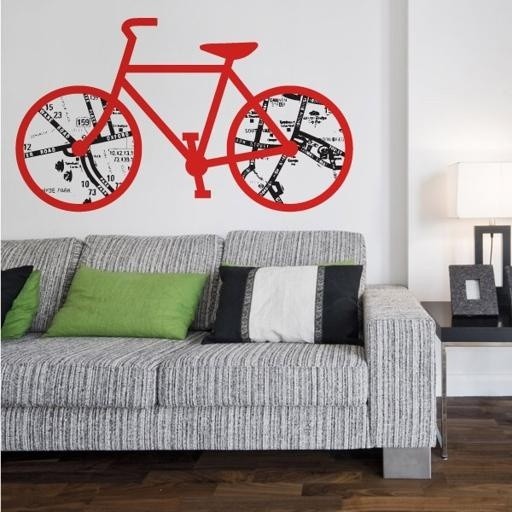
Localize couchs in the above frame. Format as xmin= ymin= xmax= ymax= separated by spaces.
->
xmin=1 ymin=284 xmax=437 ymax=480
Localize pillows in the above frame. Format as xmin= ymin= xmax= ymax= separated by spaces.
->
xmin=209 ymin=230 xmax=365 ymax=343
xmin=42 ymin=262 xmax=209 ymax=340
xmin=0 ymin=238 xmax=84 ymax=333
xmin=201 ymin=266 xmax=364 ymax=346
xmin=1 ymin=265 xmax=34 ymax=327
xmin=76 ymin=234 xmax=225 ymax=331
xmin=2 ymin=270 xmax=42 ymax=339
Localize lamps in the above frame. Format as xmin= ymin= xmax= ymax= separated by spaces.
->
xmin=445 ymin=162 xmax=512 ymax=265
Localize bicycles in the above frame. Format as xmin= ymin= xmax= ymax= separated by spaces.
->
xmin=14 ymin=16 xmax=354 ymax=212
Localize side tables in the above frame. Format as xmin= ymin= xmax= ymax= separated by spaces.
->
xmin=420 ymin=302 xmax=512 ymax=460
